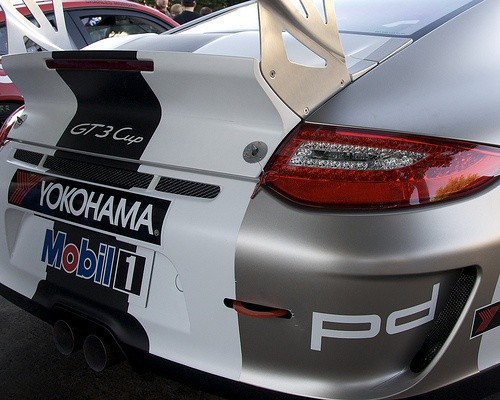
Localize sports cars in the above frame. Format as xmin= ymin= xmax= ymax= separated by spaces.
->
xmin=0 ymin=0 xmax=500 ymax=400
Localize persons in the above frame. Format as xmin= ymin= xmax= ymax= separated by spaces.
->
xmin=143 ymin=0 xmax=213 ymax=25
xmin=106 ymin=15 xmax=131 ymax=38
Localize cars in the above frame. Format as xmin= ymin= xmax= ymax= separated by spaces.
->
xmin=0 ymin=0 xmax=178 ymax=119
